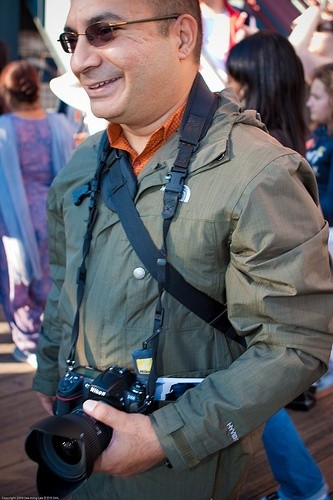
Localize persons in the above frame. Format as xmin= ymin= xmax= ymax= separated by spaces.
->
xmin=299 ymin=63 xmax=333 ymax=389
xmin=32 ymin=0 xmax=333 ymax=500
xmin=0 ymin=61 xmax=77 ymax=370
xmin=222 ymin=29 xmax=333 ymax=500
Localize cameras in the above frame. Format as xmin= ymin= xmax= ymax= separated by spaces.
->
xmin=24 ymin=366 xmax=152 ymax=482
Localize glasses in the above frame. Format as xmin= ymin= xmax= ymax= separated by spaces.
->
xmin=56 ymin=15 xmax=178 ymax=54
